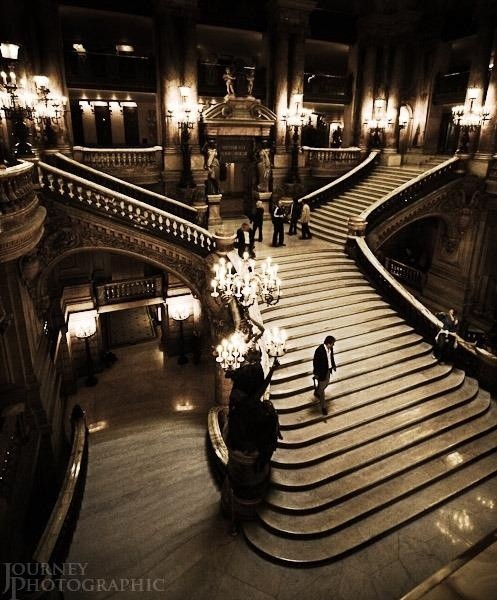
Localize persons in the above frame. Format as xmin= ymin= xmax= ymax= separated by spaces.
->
xmin=312 ymin=336 xmax=336 ymax=415
xmin=297 ymin=199 xmax=312 ymax=240
xmin=236 ymin=223 xmax=256 ymax=261
xmin=222 ymin=67 xmax=236 ymax=98
xmin=249 ymin=199 xmax=264 ymax=242
xmin=331 ymin=125 xmax=344 ymax=155
xmin=287 ymin=196 xmax=301 ymax=236
xmin=246 ymin=70 xmax=255 ymax=95
xmin=271 ymin=200 xmax=287 ymax=247
xmin=433 ymin=307 xmax=458 ymax=344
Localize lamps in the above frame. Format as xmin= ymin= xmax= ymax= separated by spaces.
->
xmin=0 ymin=42 xmax=69 ymax=159
xmin=162 ymin=81 xmax=203 ymax=187
xmin=278 ymin=89 xmax=313 ymax=183
xmin=364 ymin=93 xmax=412 ymax=152
xmin=74 ymin=316 xmax=97 ymax=387
xmin=447 ymin=79 xmax=491 ymax=153
xmin=73 ymin=43 xmax=148 ymax=60
xmin=173 ymin=306 xmax=191 ymax=365
xmin=201 ymin=252 xmax=289 ymax=400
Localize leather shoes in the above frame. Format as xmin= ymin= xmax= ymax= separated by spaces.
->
xmin=322 ymin=408 xmax=327 ymax=415
xmin=314 ymin=392 xmax=320 ymax=398
xmin=272 ymin=244 xmax=279 ymax=247
xmin=278 ymin=243 xmax=286 ymax=246
